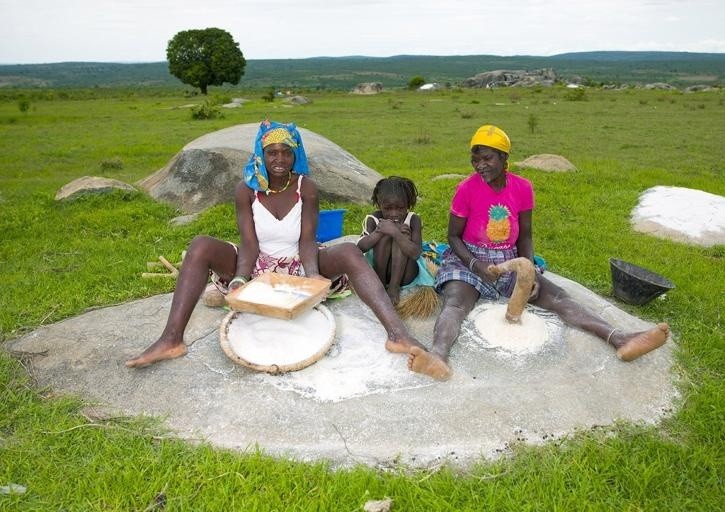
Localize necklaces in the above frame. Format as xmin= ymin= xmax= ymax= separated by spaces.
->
xmin=261 ymin=172 xmax=292 ymax=194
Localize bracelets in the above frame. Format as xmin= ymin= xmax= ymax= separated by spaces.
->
xmin=227 ymin=274 xmax=249 ymax=288
xmin=469 ymin=258 xmax=479 ymax=271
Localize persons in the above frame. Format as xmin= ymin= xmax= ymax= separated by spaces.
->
xmin=121 ymin=115 xmax=432 ymax=370
xmin=403 ymin=124 xmax=673 ymax=383
xmin=353 ymin=175 xmax=435 ymax=307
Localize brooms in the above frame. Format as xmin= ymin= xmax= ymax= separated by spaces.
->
xmin=392 ymin=285 xmax=443 ymax=323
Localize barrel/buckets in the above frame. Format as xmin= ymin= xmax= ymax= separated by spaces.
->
xmin=314 ymin=210 xmax=345 ymax=244
